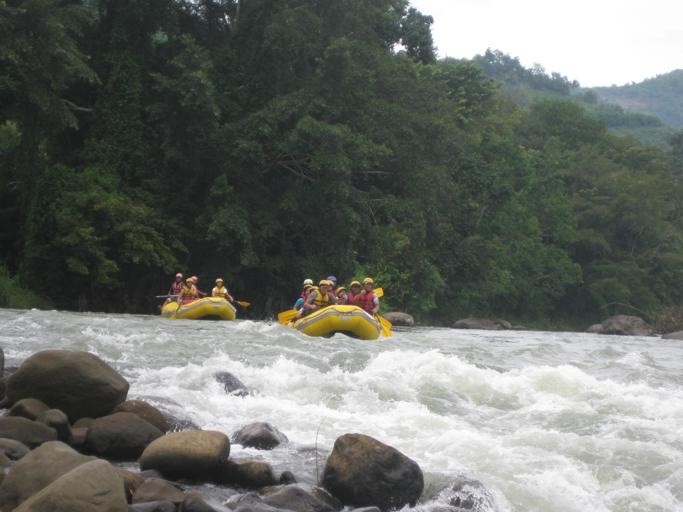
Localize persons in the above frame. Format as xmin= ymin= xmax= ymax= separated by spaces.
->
xmin=211 ymin=278 xmax=233 ymax=302
xmin=176 ymin=277 xmax=196 ymax=305
xmin=293 ymin=275 xmax=380 ymax=319
xmin=191 ymin=276 xmax=207 ymax=299
xmin=162 ymin=273 xmax=184 ymax=305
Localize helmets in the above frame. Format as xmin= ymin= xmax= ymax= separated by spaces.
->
xmin=303 ymin=278 xmax=314 ymax=285
xmin=303 ymin=284 xmax=320 ymax=294
xmin=185 ymin=278 xmax=193 ymax=284
xmin=349 ymin=280 xmax=361 ymax=290
xmin=362 ymin=278 xmax=374 ymax=285
xmin=175 ymin=272 xmax=183 ymax=278
xmin=191 ymin=275 xmax=200 ymax=281
xmin=215 ymin=278 xmax=224 ymax=284
xmin=319 ymin=279 xmax=335 ymax=288
xmin=335 ymin=287 xmax=346 ymax=298
xmin=326 ymin=275 xmax=337 ymax=282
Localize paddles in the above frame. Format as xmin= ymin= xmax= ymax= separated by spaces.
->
xmin=169 ymin=290 xmax=190 ymax=320
xmin=278 ymin=298 xmax=344 ymax=324
xmin=224 ymin=293 xmax=250 ymax=306
xmin=365 ymin=295 xmax=393 ymax=337
xmin=373 ymin=288 xmax=384 ymax=297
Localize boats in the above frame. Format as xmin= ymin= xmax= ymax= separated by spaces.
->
xmin=161 ymin=296 xmax=235 ymax=320
xmin=289 ymin=304 xmax=380 ymax=340
